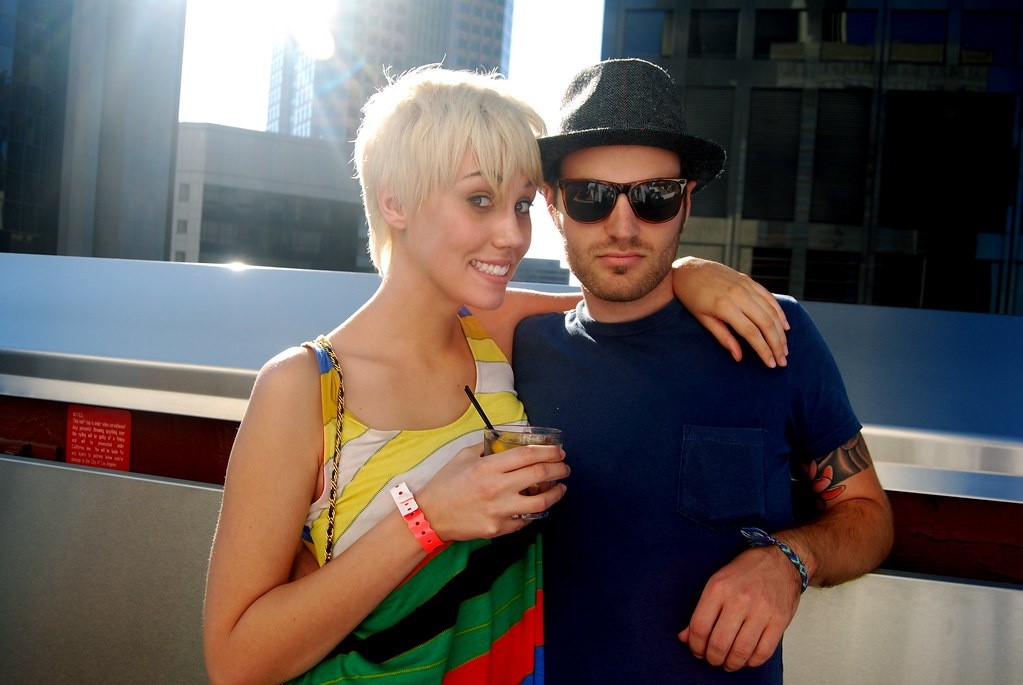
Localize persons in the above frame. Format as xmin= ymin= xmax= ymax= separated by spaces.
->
xmin=203 ymin=67 xmax=789 ymax=685
xmin=510 ymin=58 xmax=892 ymax=685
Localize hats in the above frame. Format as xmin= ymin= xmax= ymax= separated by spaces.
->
xmin=538 ymin=58 xmax=727 ymax=197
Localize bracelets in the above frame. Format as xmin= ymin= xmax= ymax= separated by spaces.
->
xmin=390 ymin=481 xmax=442 ymax=556
xmin=741 ymin=525 xmax=809 ymax=594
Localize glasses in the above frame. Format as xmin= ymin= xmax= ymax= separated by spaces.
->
xmin=554 ymin=177 xmax=690 ymax=224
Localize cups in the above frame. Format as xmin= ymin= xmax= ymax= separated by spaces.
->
xmin=483 ymin=424 xmax=563 ymax=519
